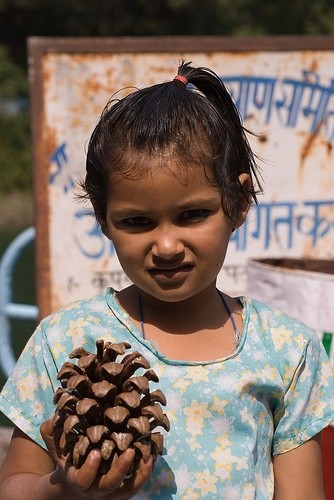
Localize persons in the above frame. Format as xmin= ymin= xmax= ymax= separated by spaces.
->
xmin=1 ymin=59 xmax=334 ymax=500
